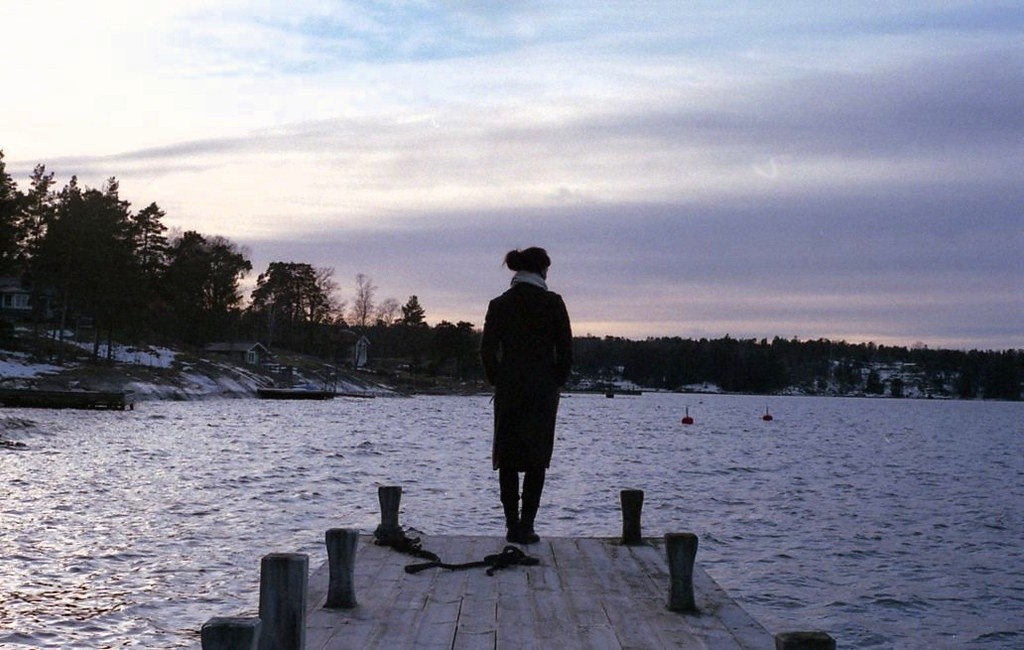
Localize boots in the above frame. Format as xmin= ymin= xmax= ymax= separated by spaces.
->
xmin=519 ymin=493 xmax=540 ymax=543
xmin=502 ymin=495 xmax=520 ymax=542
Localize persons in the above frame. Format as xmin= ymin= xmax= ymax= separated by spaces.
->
xmin=480 ymin=246 xmax=574 ymax=545
